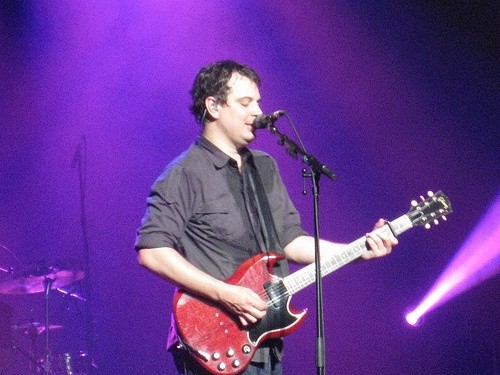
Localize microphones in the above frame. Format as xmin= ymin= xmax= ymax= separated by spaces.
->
xmin=253 ymin=110 xmax=284 ymax=129
xmin=70 ymin=134 xmax=86 ymax=169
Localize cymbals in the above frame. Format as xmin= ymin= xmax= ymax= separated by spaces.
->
xmin=8 ymin=320 xmax=62 ymax=336
xmin=0 ymin=265 xmax=85 ymax=295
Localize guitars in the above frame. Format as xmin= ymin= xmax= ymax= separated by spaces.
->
xmin=171 ymin=190 xmax=456 ymax=375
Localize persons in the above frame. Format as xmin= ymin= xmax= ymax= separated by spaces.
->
xmin=133 ymin=59 xmax=399 ymax=375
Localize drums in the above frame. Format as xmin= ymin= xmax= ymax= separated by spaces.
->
xmin=42 ymin=349 xmax=96 ymax=375
